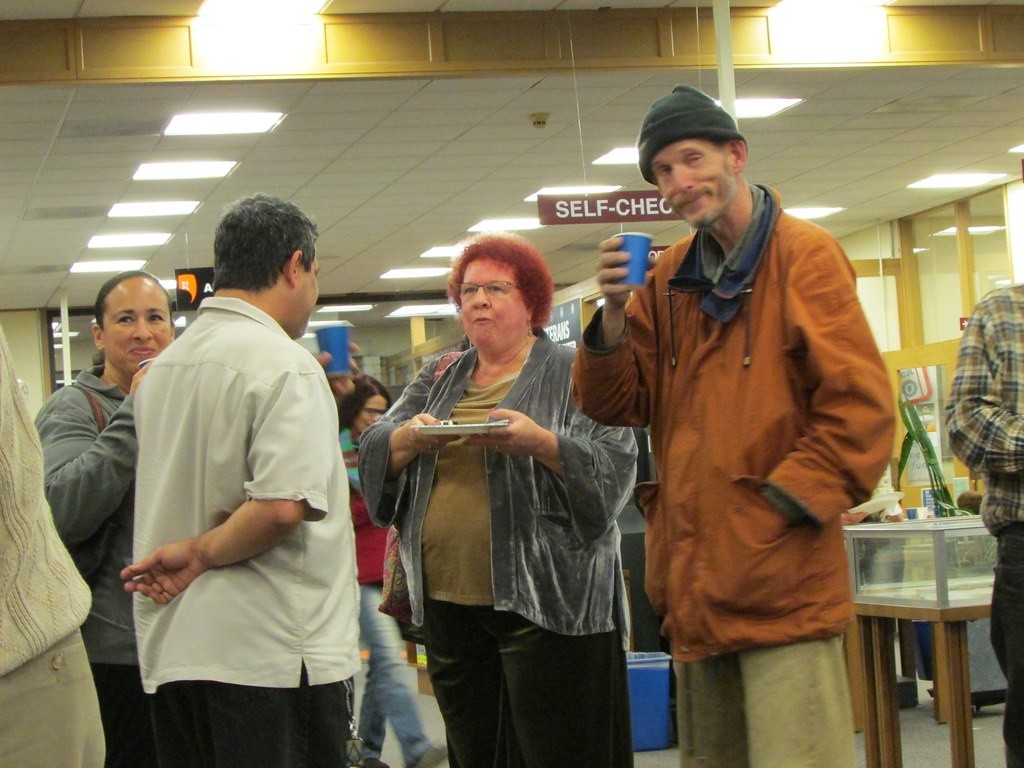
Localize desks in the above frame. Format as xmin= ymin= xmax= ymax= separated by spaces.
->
xmin=856 ymin=605 xmax=993 ymax=767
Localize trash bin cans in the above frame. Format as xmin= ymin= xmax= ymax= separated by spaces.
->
xmin=624 ymin=651 xmax=674 ymax=752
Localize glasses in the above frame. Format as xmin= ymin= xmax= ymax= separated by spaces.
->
xmin=361 ymin=408 xmax=387 ymax=417
xmin=454 ymin=280 xmax=523 ymax=300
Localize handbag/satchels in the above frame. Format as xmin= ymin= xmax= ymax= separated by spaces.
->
xmin=378 ymin=524 xmax=411 ymax=625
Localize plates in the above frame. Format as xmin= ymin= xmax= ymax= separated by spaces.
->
xmin=409 ymin=423 xmax=509 ymax=436
xmin=847 ymin=492 xmax=905 ymax=514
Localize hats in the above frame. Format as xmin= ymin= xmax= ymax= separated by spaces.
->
xmin=638 ymin=83 xmax=747 ymax=187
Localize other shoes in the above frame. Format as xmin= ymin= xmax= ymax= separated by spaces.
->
xmin=409 ymin=743 xmax=447 ymax=768
xmin=361 ymin=758 xmax=390 ymax=768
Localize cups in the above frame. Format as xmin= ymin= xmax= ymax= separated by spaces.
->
xmin=138 ymin=357 xmax=156 ymax=370
xmin=611 ymin=232 xmax=653 ymax=290
xmin=907 ymin=508 xmax=916 ymax=519
xmin=316 ymin=323 xmax=356 ymax=378
xmin=917 ymin=507 xmax=928 ymax=519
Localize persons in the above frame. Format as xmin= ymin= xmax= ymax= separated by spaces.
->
xmin=839 ymin=461 xmax=904 ymax=583
xmin=944 ymin=285 xmax=1023 ymax=768
xmin=33 ymin=269 xmax=178 ymax=768
xmin=119 ymin=192 xmax=361 ymax=768
xmin=0 ymin=327 xmax=106 ymax=768
xmin=340 ymin=372 xmax=448 ymax=768
xmin=571 ymin=83 xmax=897 ymax=768
xmin=356 ymin=229 xmax=641 ymax=766
